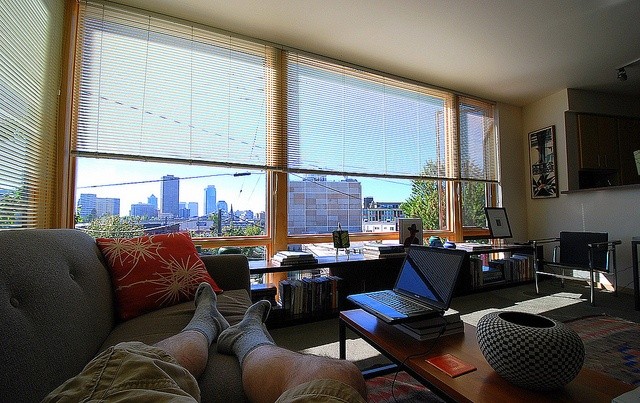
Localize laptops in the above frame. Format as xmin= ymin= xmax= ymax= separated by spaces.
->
xmin=347 ymin=244 xmax=467 ymax=325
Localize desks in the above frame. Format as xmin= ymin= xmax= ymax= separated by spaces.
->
xmin=632 ymin=237 xmax=640 ymax=311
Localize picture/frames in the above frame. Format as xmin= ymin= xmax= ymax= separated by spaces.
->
xmin=485 ymin=207 xmax=512 ymax=239
xmin=528 ymin=125 xmax=559 ymax=199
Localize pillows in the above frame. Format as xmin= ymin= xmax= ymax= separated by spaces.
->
xmin=96 ymin=232 xmax=223 ymax=320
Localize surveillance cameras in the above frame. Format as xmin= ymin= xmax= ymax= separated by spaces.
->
xmin=617 ymin=70 xmax=629 ymax=81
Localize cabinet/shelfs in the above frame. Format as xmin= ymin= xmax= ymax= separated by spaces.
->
xmin=620 ymin=116 xmax=640 ymax=185
xmin=467 ymin=245 xmax=543 ymax=294
xmin=577 ymin=113 xmax=621 ymax=187
xmin=248 ymin=257 xmax=365 ymax=326
xmin=330 ymin=252 xmax=469 ymax=311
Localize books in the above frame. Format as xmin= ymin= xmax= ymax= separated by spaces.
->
xmin=363 ymin=243 xmax=408 ymax=260
xmin=469 ymin=252 xmax=536 ymax=288
xmin=249 ymin=274 xmax=344 ymax=323
xmin=391 ymin=306 xmax=464 ymax=342
xmin=271 ymin=250 xmax=318 ymax=268
xmin=425 ymin=353 xmax=477 ymax=378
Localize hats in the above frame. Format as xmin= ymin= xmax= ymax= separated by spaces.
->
xmin=408 ymin=223 xmax=419 ymax=232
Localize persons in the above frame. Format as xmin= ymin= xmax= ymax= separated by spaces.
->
xmin=403 ymin=224 xmax=419 ymax=246
xmin=38 ymin=282 xmax=367 ymax=403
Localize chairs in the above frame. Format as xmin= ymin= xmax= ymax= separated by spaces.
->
xmin=533 ymin=232 xmax=621 ymax=304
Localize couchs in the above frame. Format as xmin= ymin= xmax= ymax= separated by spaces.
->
xmin=0 ymin=230 xmax=276 ymax=403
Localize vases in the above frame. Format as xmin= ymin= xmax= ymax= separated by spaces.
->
xmin=477 ymin=311 xmax=584 ymax=389
